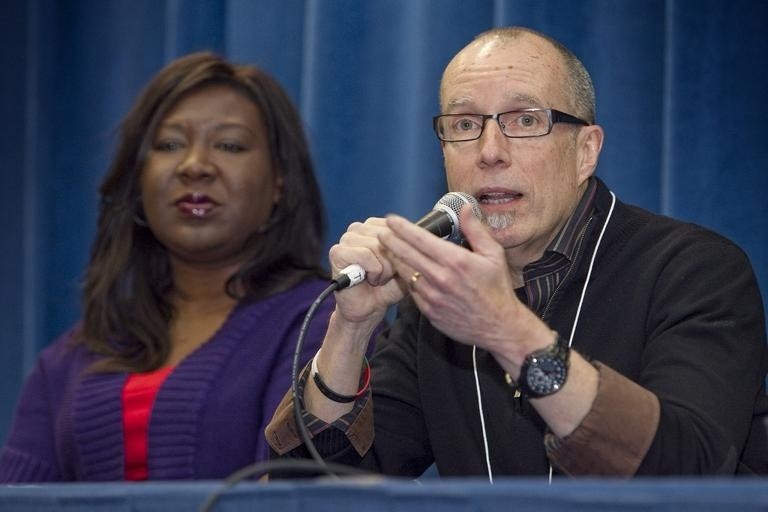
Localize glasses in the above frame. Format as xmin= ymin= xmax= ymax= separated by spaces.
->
xmin=433 ymin=105 xmax=588 ymax=142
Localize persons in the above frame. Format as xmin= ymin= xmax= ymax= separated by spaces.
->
xmin=0 ymin=52 xmax=385 ymax=488
xmin=266 ymin=23 xmax=768 ymax=480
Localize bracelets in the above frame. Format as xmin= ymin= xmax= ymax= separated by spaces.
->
xmin=313 ymin=349 xmax=372 ymax=403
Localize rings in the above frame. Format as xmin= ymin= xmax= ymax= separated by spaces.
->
xmin=409 ymin=273 xmax=422 ymax=290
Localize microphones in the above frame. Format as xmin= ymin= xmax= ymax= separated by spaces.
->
xmin=330 ymin=190 xmax=484 ymax=292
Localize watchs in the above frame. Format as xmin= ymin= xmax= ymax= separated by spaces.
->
xmin=513 ymin=331 xmax=568 ymax=396
